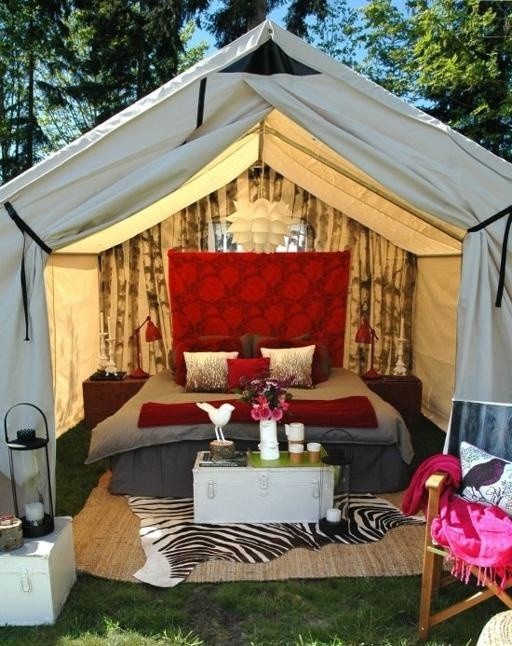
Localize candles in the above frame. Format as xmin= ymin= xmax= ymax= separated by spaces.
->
xmin=109 ymin=318 xmax=115 ymax=340
xmin=99 ymin=311 xmax=104 ymax=334
xmin=400 ymin=317 xmax=404 ymax=337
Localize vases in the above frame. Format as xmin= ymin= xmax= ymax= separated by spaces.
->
xmin=257 ymin=423 xmax=279 ymax=461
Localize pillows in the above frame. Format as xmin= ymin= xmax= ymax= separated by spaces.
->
xmin=184 ymin=351 xmax=237 ymax=392
xmin=260 ymin=345 xmax=318 ymax=387
xmin=227 ymin=359 xmax=270 ymax=389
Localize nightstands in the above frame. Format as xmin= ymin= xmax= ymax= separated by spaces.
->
xmin=83 ymin=371 xmax=150 ymax=433
xmin=363 ymin=375 xmax=422 ymax=430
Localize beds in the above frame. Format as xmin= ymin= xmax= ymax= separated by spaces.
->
xmin=85 ymin=249 xmax=417 ymax=499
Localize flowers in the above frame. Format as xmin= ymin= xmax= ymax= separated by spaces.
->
xmin=238 ymin=379 xmax=290 ymax=420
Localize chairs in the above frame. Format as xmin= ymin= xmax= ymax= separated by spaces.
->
xmin=421 ymin=457 xmax=510 ymax=625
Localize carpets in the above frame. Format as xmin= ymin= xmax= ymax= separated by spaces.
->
xmin=66 ymin=467 xmax=455 ymax=578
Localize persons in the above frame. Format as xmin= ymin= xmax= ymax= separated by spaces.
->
xmin=356 ymin=311 xmax=379 ymax=373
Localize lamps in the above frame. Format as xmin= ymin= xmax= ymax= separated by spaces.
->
xmin=129 ymin=316 xmax=160 ymax=377
xmin=353 ymin=320 xmax=377 ymax=379
xmin=225 ymin=196 xmax=296 ymax=253
